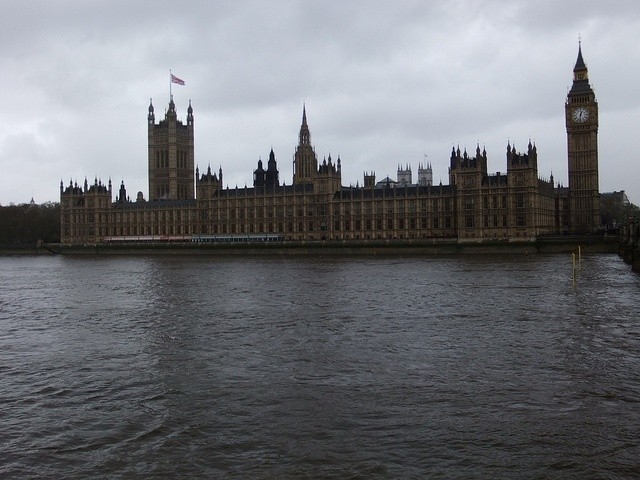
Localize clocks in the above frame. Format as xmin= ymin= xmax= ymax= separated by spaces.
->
xmin=572 ymin=107 xmax=589 ymax=123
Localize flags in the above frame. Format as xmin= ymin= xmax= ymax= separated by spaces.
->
xmin=171 ymin=74 xmax=185 ymax=86
